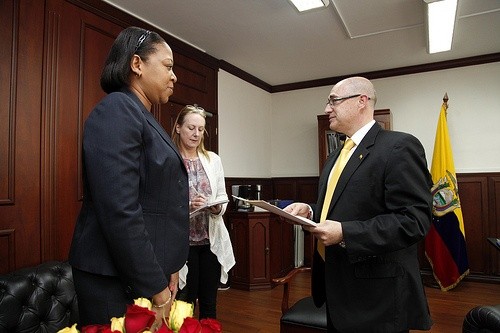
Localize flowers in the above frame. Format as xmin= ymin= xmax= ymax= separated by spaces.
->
xmin=56 ymin=297 xmax=223 ymax=333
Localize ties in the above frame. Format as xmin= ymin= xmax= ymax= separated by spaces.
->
xmin=317 ymin=139 xmax=355 ymax=262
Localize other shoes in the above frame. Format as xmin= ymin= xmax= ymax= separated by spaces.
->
xmin=217 ymin=284 xmax=230 ymax=290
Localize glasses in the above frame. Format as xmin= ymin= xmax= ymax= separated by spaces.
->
xmin=186 ymin=105 xmax=204 ymax=111
xmin=326 ymin=94 xmax=370 ymax=108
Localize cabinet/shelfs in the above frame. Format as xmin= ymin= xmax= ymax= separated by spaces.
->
xmin=230 ymin=213 xmax=293 ymax=292
xmin=317 ymin=109 xmax=392 ymax=176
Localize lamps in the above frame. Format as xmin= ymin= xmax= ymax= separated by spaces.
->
xmin=423 ymin=0 xmax=458 ymax=54
xmin=287 ymin=0 xmax=330 ymax=14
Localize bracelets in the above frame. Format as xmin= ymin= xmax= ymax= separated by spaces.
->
xmin=151 ymin=290 xmax=171 ymax=308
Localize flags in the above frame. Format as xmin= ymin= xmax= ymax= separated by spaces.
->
xmin=424 ymin=102 xmax=471 ymax=292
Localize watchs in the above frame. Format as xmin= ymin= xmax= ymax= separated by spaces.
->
xmin=339 ymin=240 xmax=346 ymax=249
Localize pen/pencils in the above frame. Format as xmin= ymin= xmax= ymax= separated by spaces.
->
xmin=192 ymin=186 xmax=208 ymax=204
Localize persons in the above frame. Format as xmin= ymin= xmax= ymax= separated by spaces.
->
xmin=67 ymin=26 xmax=190 ymax=332
xmin=278 ymin=76 xmax=434 ymax=333
xmin=169 ymin=104 xmax=236 ymax=321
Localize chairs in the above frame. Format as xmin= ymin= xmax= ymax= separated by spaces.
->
xmin=0 ymin=261 xmax=81 ymax=333
xmin=272 ymin=264 xmax=327 ymax=333
